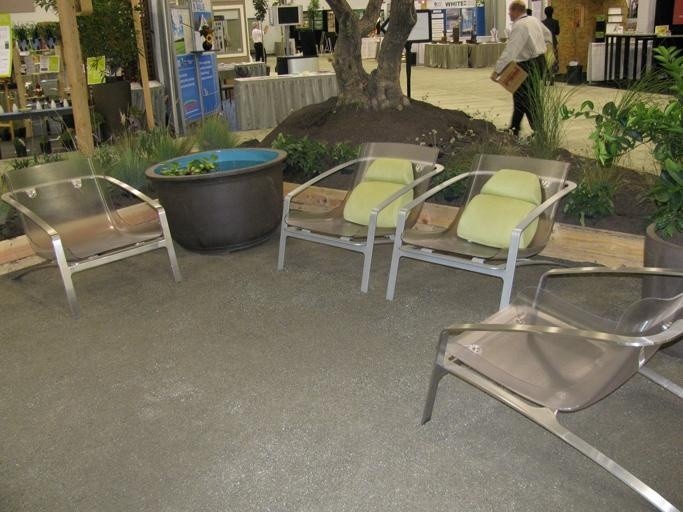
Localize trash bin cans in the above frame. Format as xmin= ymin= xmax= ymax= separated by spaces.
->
xmin=567 ymin=65 xmax=583 ymax=84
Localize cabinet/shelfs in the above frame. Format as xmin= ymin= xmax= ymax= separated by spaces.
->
xmin=12 ymin=22 xmax=75 ymax=137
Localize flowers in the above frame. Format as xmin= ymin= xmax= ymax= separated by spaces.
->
xmin=199 ymin=25 xmax=215 ymax=43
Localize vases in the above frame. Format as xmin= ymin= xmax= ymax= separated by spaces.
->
xmin=203 ymin=40 xmax=213 ymax=51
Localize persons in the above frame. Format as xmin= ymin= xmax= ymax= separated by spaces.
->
xmin=526 ymin=8 xmax=532 ymax=16
xmin=540 ymin=6 xmax=560 ymax=86
xmin=251 ymin=22 xmax=269 ymax=64
xmin=491 ymin=0 xmax=547 ymax=145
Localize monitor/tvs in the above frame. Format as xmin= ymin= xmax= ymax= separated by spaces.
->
xmin=273 ymin=5 xmax=303 ymax=27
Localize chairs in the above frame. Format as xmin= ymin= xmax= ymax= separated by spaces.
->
xmin=421 ymin=266 xmax=683 ymax=512
xmin=385 ymin=154 xmax=577 ymax=311
xmin=277 ymin=142 xmax=445 ymax=293
xmin=0 ymin=157 xmax=184 ymax=320
xmin=218 ymin=76 xmax=234 ymax=110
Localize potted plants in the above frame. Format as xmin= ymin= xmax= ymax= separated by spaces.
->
xmin=560 ymin=48 xmax=683 ymax=358
xmin=145 ymin=148 xmax=288 ymax=255
xmin=79 ymin=25 xmax=132 ymax=145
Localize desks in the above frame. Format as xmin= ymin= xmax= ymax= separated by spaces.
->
xmin=218 ymin=62 xmax=266 ymax=81
xmin=403 ymin=42 xmax=507 ymax=69
xmin=361 ymin=36 xmax=384 ymax=59
xmin=234 ymin=73 xmax=339 ymax=131
